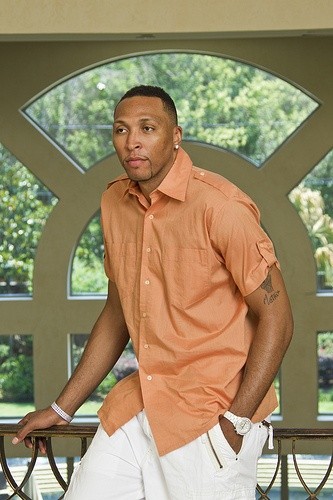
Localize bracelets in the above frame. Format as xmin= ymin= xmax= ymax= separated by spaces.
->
xmin=49 ymin=402 xmax=73 ymax=424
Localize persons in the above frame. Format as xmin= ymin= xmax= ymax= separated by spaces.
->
xmin=11 ymin=86 xmax=295 ymax=500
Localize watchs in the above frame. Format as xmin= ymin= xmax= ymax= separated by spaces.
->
xmin=222 ymin=411 xmax=252 ymax=436
